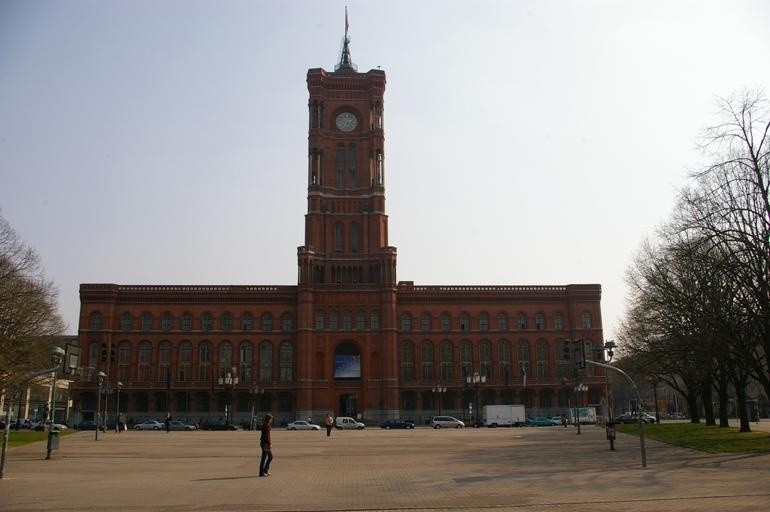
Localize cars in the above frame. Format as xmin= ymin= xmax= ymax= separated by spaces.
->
xmin=133 ymin=420 xmax=238 ymax=431
xmin=286 ymin=421 xmax=321 ymax=431
xmin=380 ymin=418 xmax=415 ymax=430
xmin=606 ymin=412 xmax=657 ymax=426
xmin=0 ymin=419 xmax=105 ymax=431
xmin=528 ymin=416 xmax=568 ymax=427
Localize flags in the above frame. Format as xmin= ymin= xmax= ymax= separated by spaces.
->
xmin=522 ymin=369 xmax=527 ymax=388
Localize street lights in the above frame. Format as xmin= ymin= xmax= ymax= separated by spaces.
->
xmin=218 ymin=372 xmax=238 ymax=423
xmin=465 ymin=371 xmax=487 ymax=427
xmin=248 ymin=381 xmax=265 ymax=431
xmin=432 ymin=383 xmax=447 ymax=416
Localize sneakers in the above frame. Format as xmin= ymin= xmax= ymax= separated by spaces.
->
xmin=259 ymin=472 xmax=271 ymax=477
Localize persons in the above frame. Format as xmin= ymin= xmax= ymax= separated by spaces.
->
xmin=165 ymin=412 xmax=173 ymax=433
xmin=259 ymin=414 xmax=273 ymax=477
xmin=119 ymin=412 xmax=126 ymax=432
xmin=324 ymin=413 xmax=333 ymax=436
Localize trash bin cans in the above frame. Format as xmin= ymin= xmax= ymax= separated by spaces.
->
xmin=48 ymin=431 xmax=61 ymax=450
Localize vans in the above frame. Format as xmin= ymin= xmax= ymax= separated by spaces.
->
xmin=431 ymin=415 xmax=466 ymax=429
xmin=335 ymin=417 xmax=365 ymax=430
